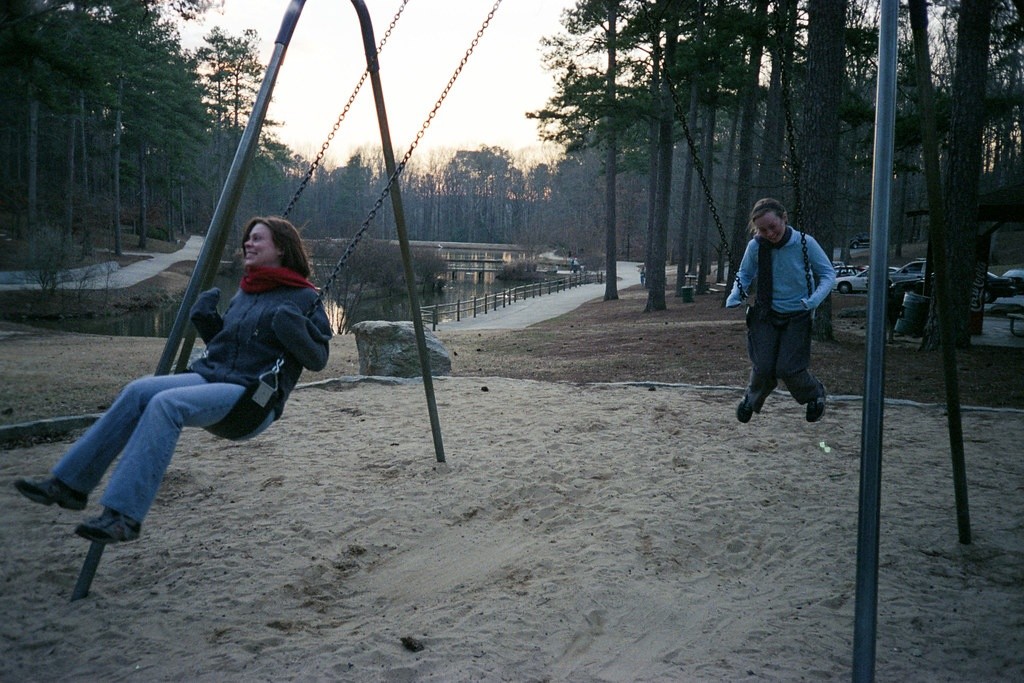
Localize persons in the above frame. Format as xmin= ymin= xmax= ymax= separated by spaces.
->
xmin=640 ymin=269 xmax=645 ymax=286
xmin=14 ymin=218 xmax=334 ymax=545
xmin=725 ymin=199 xmax=836 ymax=423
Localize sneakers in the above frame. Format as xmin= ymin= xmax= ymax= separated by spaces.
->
xmin=13 ymin=477 xmax=87 ymax=511
xmin=736 ymin=393 xmax=753 ymax=423
xmin=74 ymin=506 xmax=141 ymax=544
xmin=806 ymin=385 xmax=827 ymax=422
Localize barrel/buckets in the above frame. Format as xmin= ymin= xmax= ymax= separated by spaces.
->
xmin=894 ymin=291 xmax=932 ymax=338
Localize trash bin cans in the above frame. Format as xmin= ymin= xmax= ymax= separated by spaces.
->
xmin=681 ymin=285 xmax=697 ymax=303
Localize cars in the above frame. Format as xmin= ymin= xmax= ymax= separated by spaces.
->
xmin=1002 ymin=268 xmax=1024 ymax=295
xmin=849 ymin=233 xmax=870 ymax=249
xmin=866 ymin=260 xmax=925 ymax=290
xmin=833 ymin=260 xmax=845 ymax=269
xmin=833 ymin=264 xmax=868 ymax=277
xmin=832 ymin=267 xmax=901 ymax=295
xmin=889 ymin=270 xmax=1017 ymax=304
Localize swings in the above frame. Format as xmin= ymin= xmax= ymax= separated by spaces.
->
xmin=189 ymin=0 xmax=502 ymax=444
xmin=632 ymin=0 xmax=815 ymax=372
